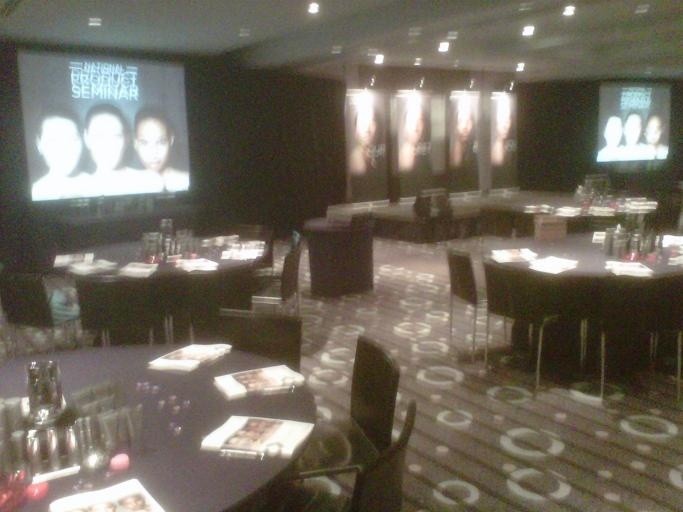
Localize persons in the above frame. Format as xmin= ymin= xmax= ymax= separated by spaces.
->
xmin=75 ymin=101 xmax=138 ymax=200
xmin=232 ymin=368 xmax=272 ymax=391
xmin=489 ymin=95 xmax=510 ymax=164
xmin=225 ymin=417 xmax=278 ymax=448
xmin=618 ymin=111 xmax=654 ymax=163
xmin=345 ymin=89 xmax=393 ymax=177
xmin=594 ymin=115 xmax=628 ymax=165
xmin=397 ymin=92 xmax=430 ymax=173
xmin=29 ymin=105 xmax=96 ymax=205
xmin=114 ymin=492 xmax=149 ymax=511
xmin=643 ymin=110 xmax=668 ymax=161
xmin=128 ymin=106 xmax=190 ymax=194
xmin=99 ymin=500 xmax=116 ymax=511
xmin=448 ymin=98 xmax=475 ymax=169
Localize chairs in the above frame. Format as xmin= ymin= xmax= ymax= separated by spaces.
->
xmin=2 ymin=223 xmax=418 ymax=511
xmin=442 ymin=216 xmax=683 ymax=403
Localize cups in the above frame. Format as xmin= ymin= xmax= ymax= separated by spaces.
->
xmin=141 ymin=218 xmax=197 ymax=264
xmin=0 ymin=360 xmax=102 ymax=476
xmin=605 ymin=227 xmax=654 ymax=257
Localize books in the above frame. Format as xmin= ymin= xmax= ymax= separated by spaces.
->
xmin=196 ymin=409 xmax=314 ymax=461
xmin=210 ymin=361 xmax=306 ymax=400
xmin=116 ymin=261 xmax=159 ymax=279
xmin=148 ymin=340 xmax=232 ymax=375
xmin=526 ymin=194 xmax=659 ymax=217
xmin=173 ymin=256 xmax=219 ymax=273
xmin=487 ymin=245 xmax=541 ymax=266
xmin=45 ymin=474 xmax=168 ymax=512
xmin=51 ymin=252 xmax=95 ymax=271
xmin=233 ymin=240 xmax=265 ymax=257
xmin=64 ymin=258 xmax=118 ymax=277
xmin=605 ymin=259 xmax=653 ymax=278
xmin=592 ymin=230 xmax=608 ymax=244
xmin=218 ymin=248 xmax=259 ymax=262
xmin=202 ymin=235 xmax=240 ymax=247
xmin=526 ymin=254 xmax=579 ymax=275
